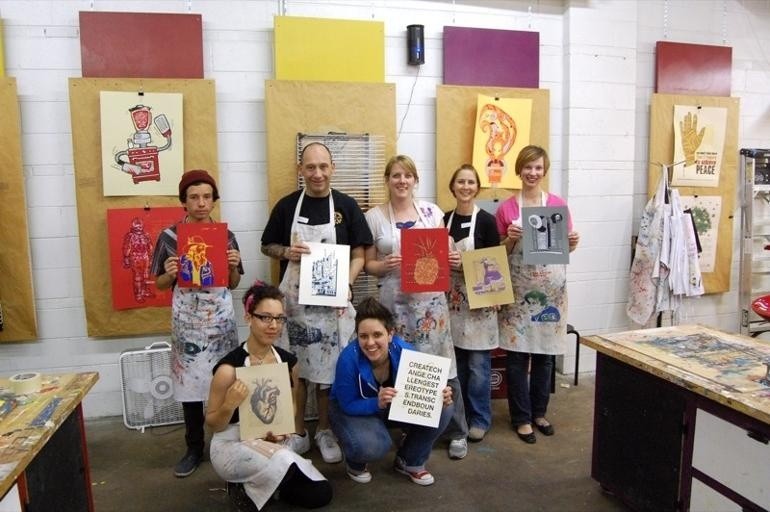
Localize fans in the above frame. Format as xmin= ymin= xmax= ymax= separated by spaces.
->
xmin=116 ymin=342 xmax=205 ymax=435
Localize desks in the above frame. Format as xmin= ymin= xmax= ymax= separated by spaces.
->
xmin=579 ymin=325 xmax=770 ymax=512
xmin=0 ymin=371 xmax=101 ymax=511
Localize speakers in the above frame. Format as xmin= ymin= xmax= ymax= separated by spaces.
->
xmin=407 ymin=23 xmax=425 ymax=66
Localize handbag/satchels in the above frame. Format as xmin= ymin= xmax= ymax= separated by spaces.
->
xmin=668 ymin=189 xmax=706 ymax=296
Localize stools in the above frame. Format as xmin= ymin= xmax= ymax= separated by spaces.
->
xmin=551 ymin=325 xmax=580 ymax=393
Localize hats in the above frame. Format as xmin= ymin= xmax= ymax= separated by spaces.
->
xmin=179 ymin=170 xmax=217 ymax=196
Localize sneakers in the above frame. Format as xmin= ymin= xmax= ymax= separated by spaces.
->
xmin=282 ymin=429 xmax=342 ymax=463
xmin=449 ymin=418 xmax=553 ymax=459
xmin=394 ymin=456 xmax=434 ymax=486
xmin=175 ymin=452 xmax=203 ymax=477
xmin=346 ymin=466 xmax=372 ymax=484
xmin=228 ymin=482 xmax=254 ymax=510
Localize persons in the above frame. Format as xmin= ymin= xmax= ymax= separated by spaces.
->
xmin=261 ymin=142 xmax=374 ymax=464
xmin=443 ymin=164 xmax=501 ymax=442
xmin=327 ymin=297 xmax=454 ymax=486
xmin=150 ymin=169 xmax=245 ymax=478
xmin=363 ymin=155 xmax=470 ymax=459
xmin=205 ymin=280 xmax=333 ymax=512
xmin=496 ymin=145 xmax=579 ymax=444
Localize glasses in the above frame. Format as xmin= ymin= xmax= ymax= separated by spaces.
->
xmin=251 ymin=313 xmax=287 ymax=323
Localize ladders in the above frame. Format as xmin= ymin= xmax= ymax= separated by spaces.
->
xmin=740 ymin=148 xmax=770 ymax=338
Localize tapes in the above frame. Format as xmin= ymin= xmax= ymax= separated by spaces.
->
xmin=8 ymin=373 xmax=41 ymax=394
